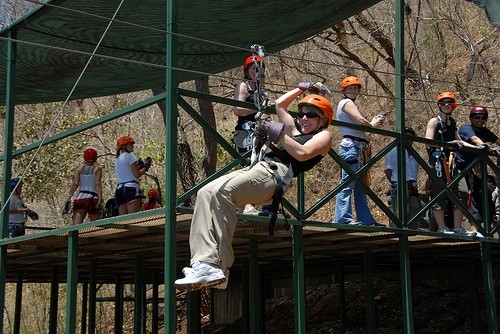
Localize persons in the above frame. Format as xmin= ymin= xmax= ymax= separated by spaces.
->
xmin=104 ymin=188 xmax=162 ymax=218
xmin=65 ymin=149 xmax=102 ymax=226
xmin=114 ymin=136 xmax=152 ymax=215
xmin=172 ymin=82 xmax=333 ymax=290
xmin=383 ymin=128 xmax=420 ymax=230
xmin=181 ymin=197 xmax=194 ymax=209
xmin=333 ymin=76 xmax=386 ymax=228
xmin=424 ymin=92 xmax=500 ymax=238
xmin=232 ymin=56 xmax=266 ymax=215
xmin=7 ymin=178 xmax=38 ymax=239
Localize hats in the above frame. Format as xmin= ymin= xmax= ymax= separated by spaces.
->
xmin=405 ymin=127 xmax=416 ymax=135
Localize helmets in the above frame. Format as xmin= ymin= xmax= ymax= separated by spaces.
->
xmin=117 ymin=136 xmax=135 ymax=149
xmin=470 ymin=106 xmax=488 ymax=119
xmin=149 ymin=189 xmax=158 ymax=198
xmin=297 ymin=94 xmax=334 ymax=125
xmin=342 ymin=76 xmax=361 ymax=91
xmin=438 ymin=92 xmax=457 ymax=110
xmin=84 ymin=149 xmax=98 ymax=162
xmin=11 ymin=178 xmax=24 ymax=187
xmin=244 ymin=56 xmax=265 ymax=78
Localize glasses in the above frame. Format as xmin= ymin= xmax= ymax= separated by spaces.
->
xmin=298 ymin=112 xmax=320 ymax=118
xmin=475 ymin=115 xmax=487 ymax=120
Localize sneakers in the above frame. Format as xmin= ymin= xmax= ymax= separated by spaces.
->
xmin=436 ymin=226 xmax=478 ymax=236
xmin=174 ymin=264 xmax=229 ymax=290
xmin=347 ymin=220 xmax=387 ymax=227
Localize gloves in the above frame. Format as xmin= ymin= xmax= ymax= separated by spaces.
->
xmin=30 ymin=211 xmax=39 ymax=220
xmin=144 ymin=157 xmax=152 ymax=172
xmin=299 ymin=81 xmax=331 ymax=95
